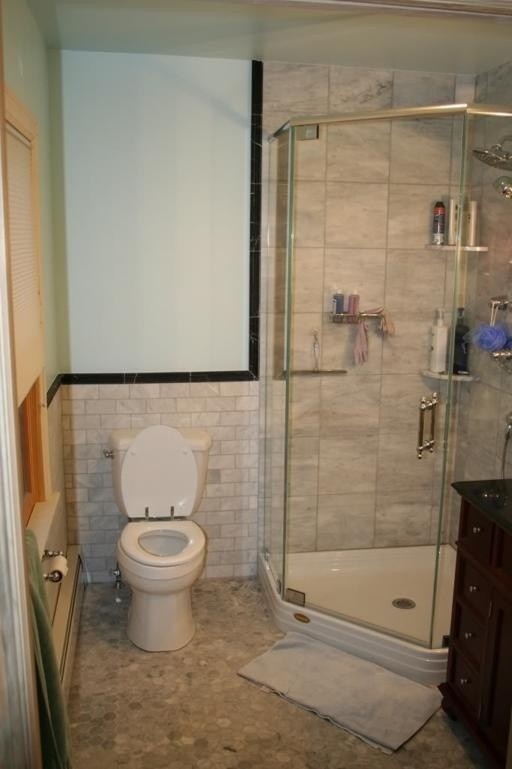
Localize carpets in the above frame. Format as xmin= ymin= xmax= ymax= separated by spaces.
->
xmin=237 ymin=631 xmax=440 ymax=756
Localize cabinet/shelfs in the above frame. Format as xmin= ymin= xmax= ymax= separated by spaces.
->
xmin=436 ymin=476 xmax=510 ymax=769
xmin=417 ymin=242 xmax=488 ymax=382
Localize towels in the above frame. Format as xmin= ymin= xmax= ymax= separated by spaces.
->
xmin=22 ymin=528 xmax=75 ymax=769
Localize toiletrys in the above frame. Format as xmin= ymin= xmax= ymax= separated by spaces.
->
xmin=347 ymin=289 xmax=360 ymax=314
xmin=331 ymin=286 xmax=345 ymax=314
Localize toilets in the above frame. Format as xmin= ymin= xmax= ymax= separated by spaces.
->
xmin=108 ymin=423 xmax=212 ymax=652
